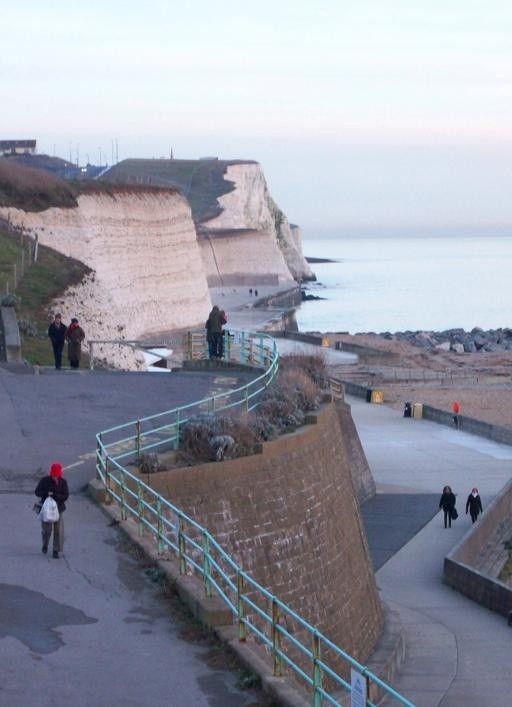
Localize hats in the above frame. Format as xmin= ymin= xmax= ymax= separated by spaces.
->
xmin=50 ymin=462 xmax=63 ymax=478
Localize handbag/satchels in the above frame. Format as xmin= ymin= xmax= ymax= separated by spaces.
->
xmin=451 ymin=508 xmax=458 ymax=520
xmin=39 ymin=495 xmax=60 ymax=522
xmin=34 ymin=502 xmax=43 ymax=514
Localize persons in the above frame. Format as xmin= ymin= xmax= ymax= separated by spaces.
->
xmin=439 ymin=485 xmax=456 ymax=528
xmin=466 ymin=488 xmax=483 ymax=525
xmin=48 ymin=313 xmax=67 ymax=370
xmin=34 ymin=463 xmax=69 ymax=559
xmin=65 ymin=318 xmax=85 ymax=368
xmin=205 ymin=305 xmax=227 ymax=358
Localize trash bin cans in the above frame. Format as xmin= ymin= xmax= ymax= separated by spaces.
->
xmin=413 ymin=403 xmax=423 ymax=419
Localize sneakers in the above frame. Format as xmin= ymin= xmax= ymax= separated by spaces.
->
xmin=211 ymin=354 xmax=225 ymax=360
xmin=42 ymin=545 xmax=59 ymax=559
xmin=56 ymin=365 xmax=80 ymax=370
